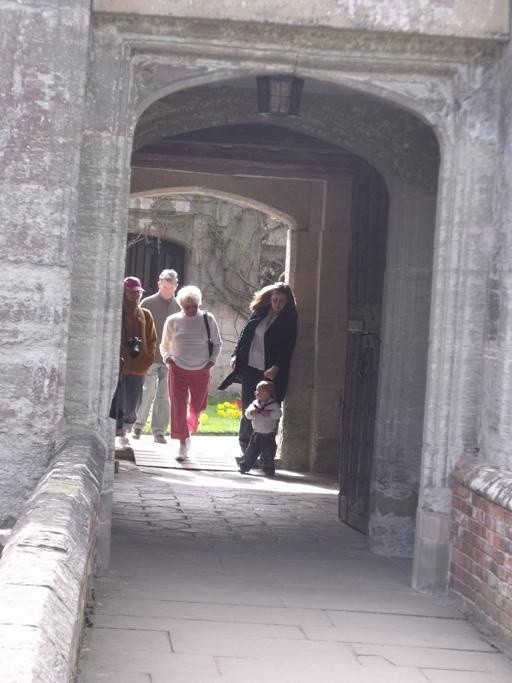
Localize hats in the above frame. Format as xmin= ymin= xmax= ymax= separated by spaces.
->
xmin=122 ymin=275 xmax=145 ymax=293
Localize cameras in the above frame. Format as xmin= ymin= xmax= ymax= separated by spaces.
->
xmin=128 ymin=336 xmax=140 ymax=357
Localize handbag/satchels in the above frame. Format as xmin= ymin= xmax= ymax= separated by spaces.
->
xmin=203 ymin=310 xmax=213 ymax=355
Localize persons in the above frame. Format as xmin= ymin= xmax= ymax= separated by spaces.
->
xmin=238 ymin=381 xmax=281 ymax=475
xmin=130 ymin=269 xmax=181 ymax=444
xmin=109 ymin=276 xmax=157 ymax=438
xmin=218 ymin=282 xmax=298 ymax=469
xmin=159 ymin=285 xmax=223 ymax=461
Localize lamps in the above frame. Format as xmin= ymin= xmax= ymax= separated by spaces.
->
xmin=256 ymin=75 xmax=304 ymax=118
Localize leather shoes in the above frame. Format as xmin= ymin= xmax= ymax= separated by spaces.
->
xmin=153 ymin=434 xmax=168 ymax=444
xmin=175 ymin=454 xmax=187 ymax=461
xmin=131 ymin=426 xmax=141 ymax=439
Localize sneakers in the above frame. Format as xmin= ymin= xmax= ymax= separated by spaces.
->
xmin=124 ymin=437 xmax=132 ymax=450
xmin=266 ymin=474 xmax=276 ymax=479
xmin=240 ymin=460 xmax=248 ymax=473
xmin=114 ymin=435 xmax=126 ymax=452
